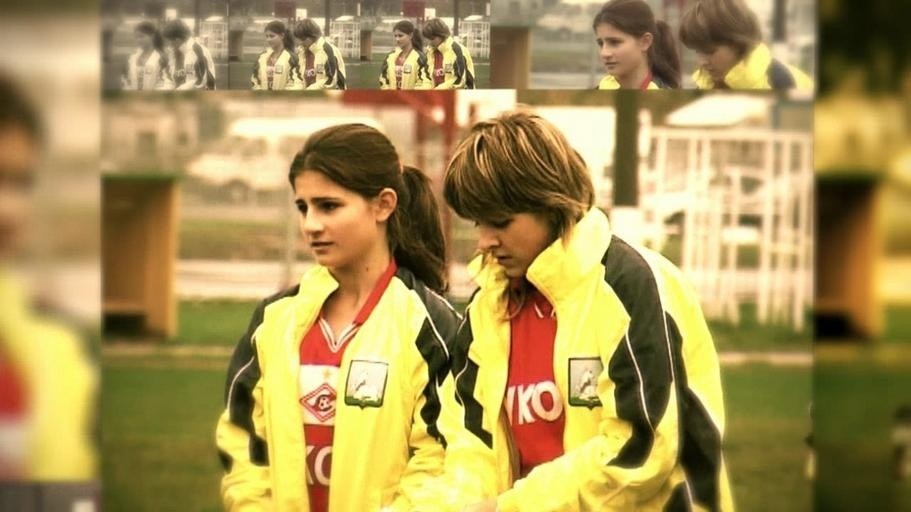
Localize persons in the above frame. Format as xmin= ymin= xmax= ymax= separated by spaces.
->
xmin=442 ymin=114 xmax=734 ymax=511
xmin=121 ymin=21 xmax=168 ymax=93
xmin=250 ymin=21 xmax=297 ymax=91
xmin=378 ymin=20 xmax=424 ymax=89
xmin=586 ymin=0 xmax=681 ymax=90
xmin=161 ymin=20 xmax=216 ymax=93
xmin=0 ymin=69 xmax=104 ymax=511
xmin=289 ymin=18 xmax=346 ymax=89
xmin=680 ymin=1 xmax=813 ymax=92
xmin=215 ymin=125 xmax=465 ymax=510
xmin=420 ymin=18 xmax=476 ymax=89
